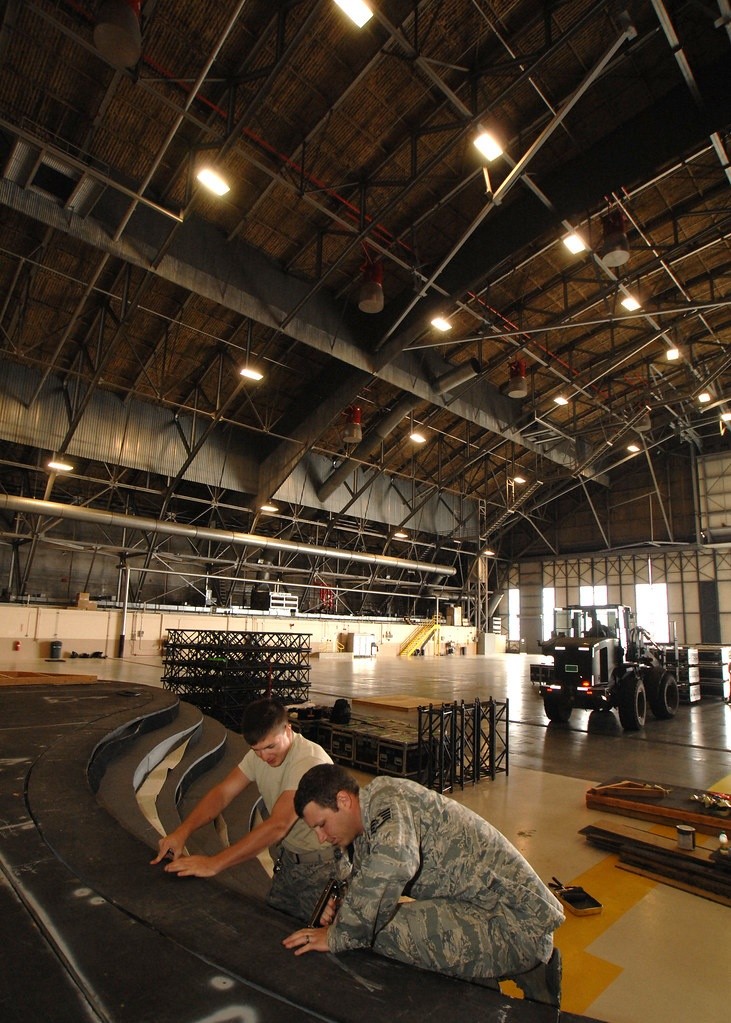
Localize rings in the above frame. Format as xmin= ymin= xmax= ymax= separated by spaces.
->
xmin=306 ymin=935 xmax=309 ymax=943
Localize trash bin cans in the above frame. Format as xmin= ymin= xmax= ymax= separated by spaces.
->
xmin=50 ymin=641 xmax=62 ymax=658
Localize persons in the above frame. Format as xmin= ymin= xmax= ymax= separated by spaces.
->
xmin=148 ymin=699 xmax=354 ymax=923
xmin=281 ymin=763 xmax=565 ymax=977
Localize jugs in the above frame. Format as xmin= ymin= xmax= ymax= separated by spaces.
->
xmin=676 ymin=825 xmax=696 ymax=850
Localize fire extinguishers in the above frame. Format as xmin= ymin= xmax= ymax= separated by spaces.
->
xmin=16 ymin=642 xmax=21 ymax=651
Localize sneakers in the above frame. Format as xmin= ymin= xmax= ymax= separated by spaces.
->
xmin=513 ymin=947 xmax=562 ymax=1008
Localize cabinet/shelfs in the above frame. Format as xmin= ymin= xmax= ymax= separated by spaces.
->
xmin=418 ymin=695 xmax=509 ymax=794
xmin=347 ymin=633 xmax=373 ymax=656
xmin=160 ymin=628 xmax=313 ymax=728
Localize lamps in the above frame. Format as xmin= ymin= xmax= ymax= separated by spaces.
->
xmin=358 ymin=265 xmax=384 ymax=313
xmin=48 ymin=458 xmax=74 ymax=472
xmin=239 ymin=367 xmax=263 ymax=381
xmin=91 ymin=1 xmax=142 ymax=67
xmin=474 ymin=132 xmax=503 ymax=161
xmin=394 ymin=531 xmax=407 ymax=538
xmin=431 ymin=316 xmax=452 ymax=331
xmin=603 ymin=211 xmax=630 ymax=268
xmin=621 ymin=296 xmax=641 ymax=311
xmin=194 ymin=166 xmax=231 ymax=197
xmin=507 ymin=361 xmax=528 ymax=397
xmin=411 ymin=432 xmax=425 ymax=445
xmin=698 ymin=393 xmax=712 ymax=402
xmin=666 ymin=349 xmax=679 ymax=360
xmin=722 ymin=413 xmax=731 ymax=421
xmin=563 ymin=234 xmax=586 ymax=254
xmin=554 ymin=396 xmax=568 ymax=406
xmin=483 ymin=548 xmax=496 ymax=557
xmin=513 ymin=477 xmax=528 ymax=484
xmin=332 ymin=1 xmax=374 ymax=29
xmin=626 ymin=446 xmax=640 ymax=453
xmin=261 ymin=503 xmax=277 ymax=515
xmin=343 ymin=406 xmax=363 ymax=444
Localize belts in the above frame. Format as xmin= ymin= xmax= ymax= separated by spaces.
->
xmin=285 ymin=846 xmax=335 ymax=864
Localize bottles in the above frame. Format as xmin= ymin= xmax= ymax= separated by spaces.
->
xmin=719 ymin=831 xmax=728 ymax=855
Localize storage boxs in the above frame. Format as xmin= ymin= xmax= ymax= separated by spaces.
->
xmin=649 ymin=646 xmax=731 ymax=704
xmin=289 ymin=706 xmax=447 ymax=779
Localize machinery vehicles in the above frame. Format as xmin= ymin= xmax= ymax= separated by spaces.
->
xmin=536 ymin=603 xmax=680 ymax=732
